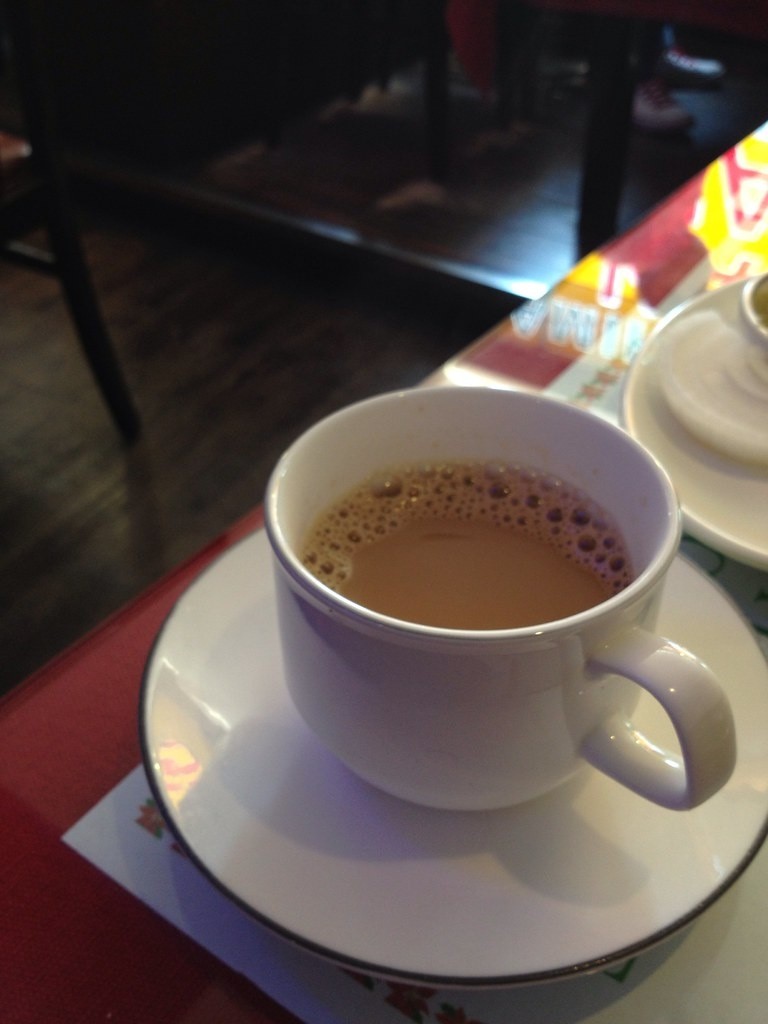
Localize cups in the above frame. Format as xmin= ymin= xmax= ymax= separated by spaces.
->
xmin=735 ymin=271 xmax=768 ymax=404
xmin=264 ymin=384 xmax=736 ymax=811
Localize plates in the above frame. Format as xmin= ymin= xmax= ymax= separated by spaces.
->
xmin=143 ymin=522 xmax=768 ymax=987
xmin=619 ymin=273 xmax=768 ymax=572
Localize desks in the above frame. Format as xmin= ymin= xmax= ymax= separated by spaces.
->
xmin=0 ymin=118 xmax=768 ymax=1024
